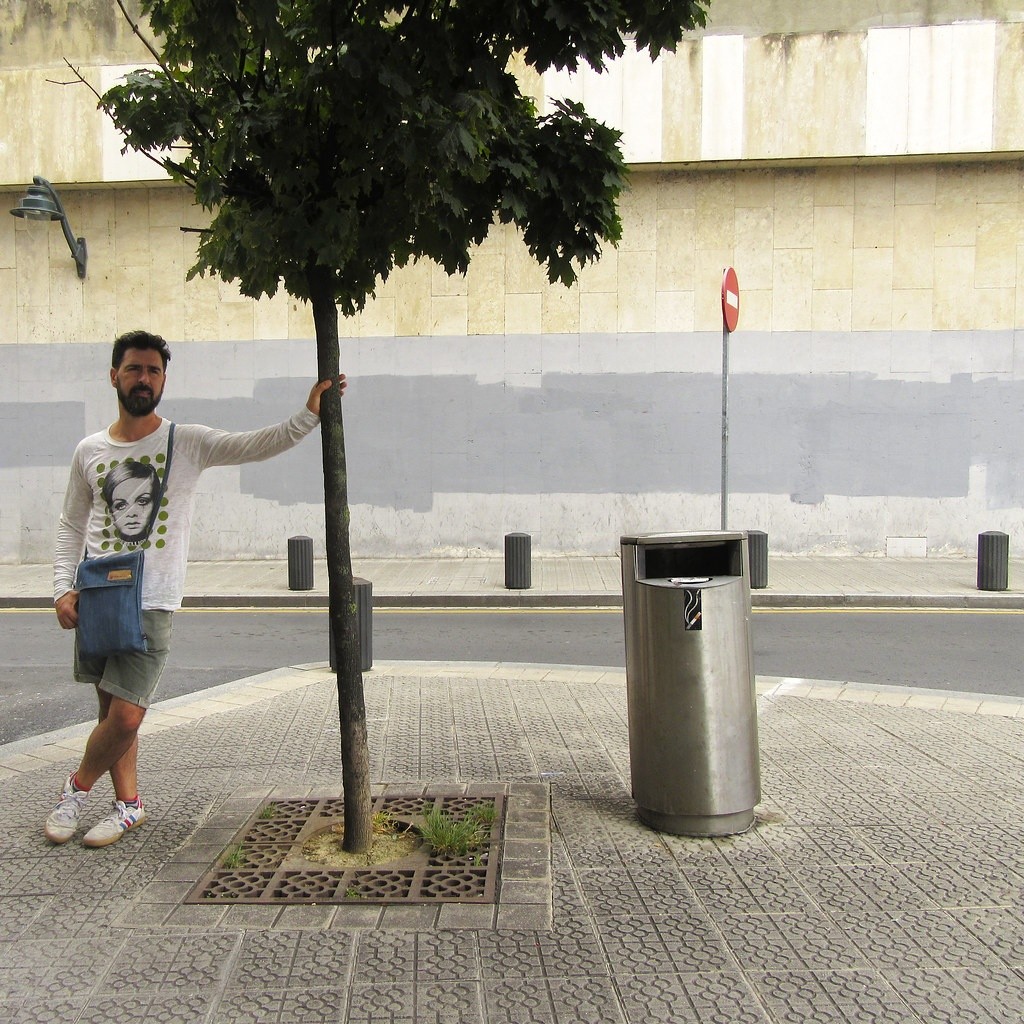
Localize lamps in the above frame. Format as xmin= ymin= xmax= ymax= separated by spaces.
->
xmin=7 ymin=175 xmax=86 ymax=281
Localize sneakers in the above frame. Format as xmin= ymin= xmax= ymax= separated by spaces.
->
xmin=82 ymin=798 xmax=146 ymax=848
xmin=44 ymin=769 xmax=90 ymax=844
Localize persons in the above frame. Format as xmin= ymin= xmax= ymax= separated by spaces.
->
xmin=46 ymin=330 xmax=347 ymax=848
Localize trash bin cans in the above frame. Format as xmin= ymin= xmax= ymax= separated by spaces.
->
xmin=617 ymin=527 xmax=765 ymax=838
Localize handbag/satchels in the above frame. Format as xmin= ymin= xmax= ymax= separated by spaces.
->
xmin=74 ymin=550 xmax=149 ymax=662
